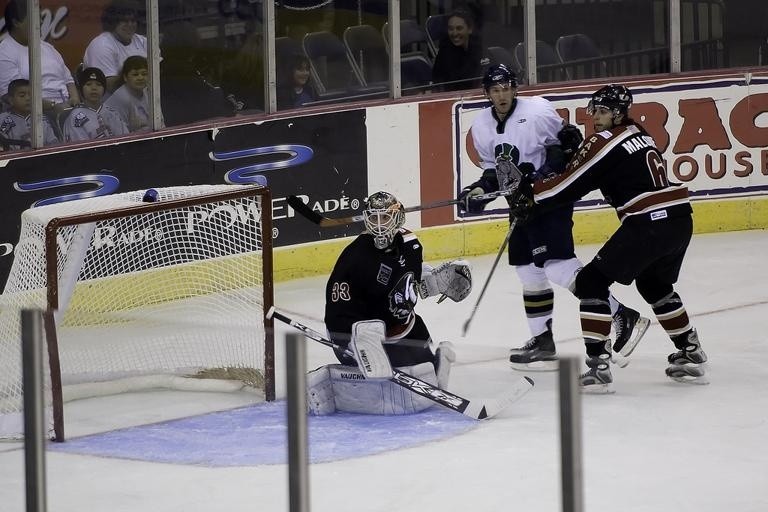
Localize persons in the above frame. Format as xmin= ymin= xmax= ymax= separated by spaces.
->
xmin=433 ymin=8 xmax=497 ymax=93
xmin=275 ymin=54 xmax=321 ymax=112
xmin=461 ymin=63 xmax=650 ymax=371
xmin=0 ymin=3 xmax=237 ymax=154
xmin=307 ymin=191 xmax=473 ymax=416
xmin=511 ymin=85 xmax=710 ymax=394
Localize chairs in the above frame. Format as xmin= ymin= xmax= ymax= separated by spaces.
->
xmin=56 ymin=108 xmax=74 ymax=141
xmin=275 ymin=14 xmax=595 ymax=96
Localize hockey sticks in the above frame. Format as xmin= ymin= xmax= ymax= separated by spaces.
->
xmin=265 ymin=307 xmax=533 ymax=419
xmin=287 ymin=189 xmax=516 ymax=227
xmin=462 ymin=220 xmax=516 ymax=334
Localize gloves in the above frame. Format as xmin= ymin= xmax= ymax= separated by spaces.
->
xmin=511 ymin=194 xmax=536 ymax=218
xmin=459 ymin=182 xmax=494 ymax=214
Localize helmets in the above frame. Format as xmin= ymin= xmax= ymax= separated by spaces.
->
xmin=591 ymin=84 xmax=633 ymax=115
xmin=362 ymin=192 xmax=406 ymax=248
xmin=483 ymin=64 xmax=516 ymax=89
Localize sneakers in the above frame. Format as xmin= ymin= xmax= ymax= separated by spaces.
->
xmin=510 ymin=343 xmax=555 ymax=363
xmin=577 ymin=305 xmax=705 ymax=387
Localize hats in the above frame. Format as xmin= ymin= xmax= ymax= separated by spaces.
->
xmin=81 ymin=67 xmax=107 ymax=96
